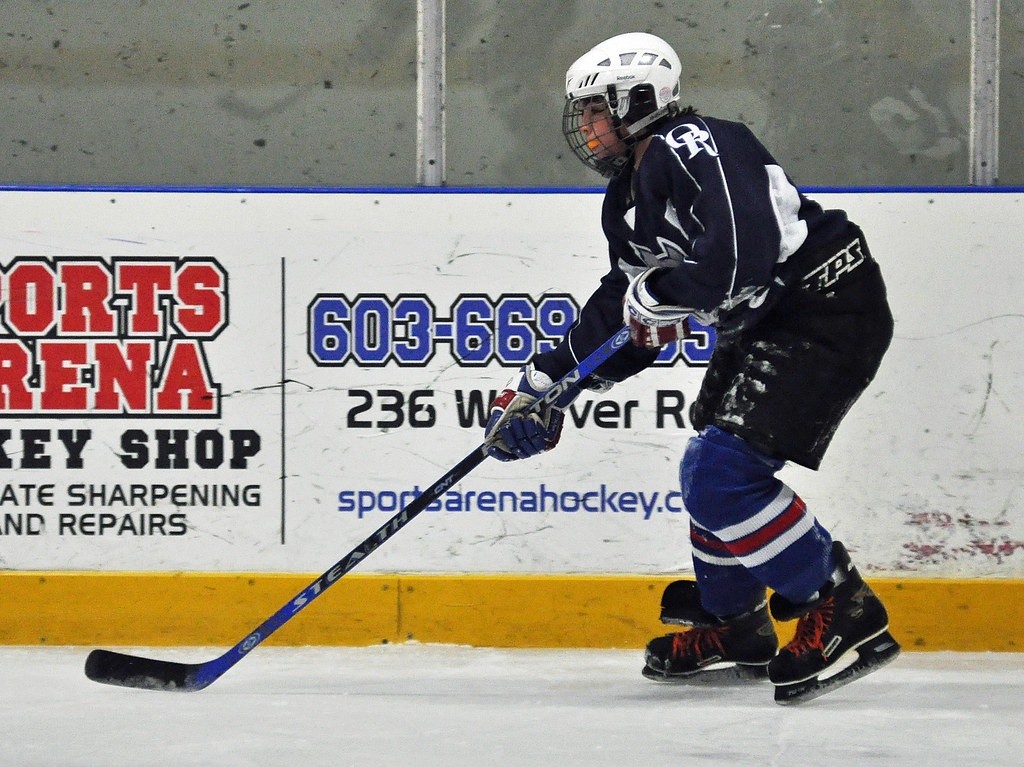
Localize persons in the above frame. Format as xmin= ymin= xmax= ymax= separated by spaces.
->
xmin=743 ymin=0 xmax=968 ymax=186
xmin=486 ymin=33 xmax=902 ymax=705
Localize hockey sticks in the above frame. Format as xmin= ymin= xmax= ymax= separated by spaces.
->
xmin=83 ymin=320 xmax=634 ymax=693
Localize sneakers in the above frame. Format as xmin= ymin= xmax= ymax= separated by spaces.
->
xmin=642 ymin=579 xmax=778 ymax=686
xmin=766 ymin=539 xmax=900 ymax=705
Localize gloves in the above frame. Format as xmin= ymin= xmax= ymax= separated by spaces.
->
xmin=622 ymin=267 xmax=691 ymax=348
xmin=484 ymin=351 xmax=582 ymax=462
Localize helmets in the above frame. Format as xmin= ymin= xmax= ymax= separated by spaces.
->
xmin=561 ymin=31 xmax=682 ymax=180
